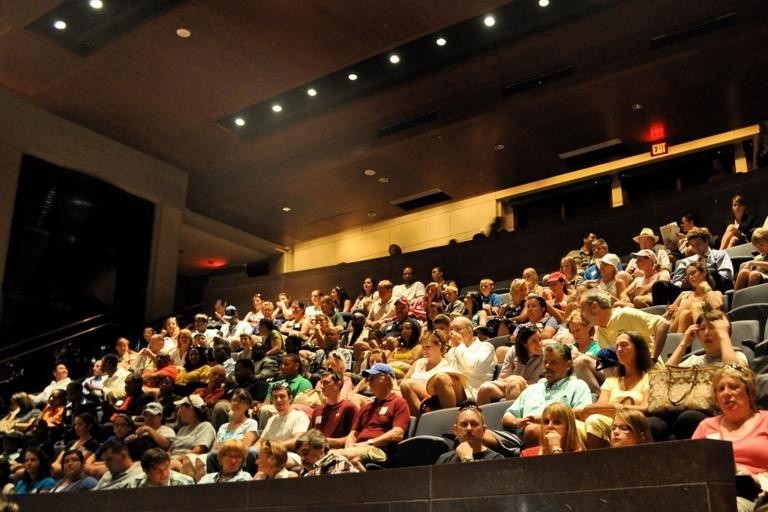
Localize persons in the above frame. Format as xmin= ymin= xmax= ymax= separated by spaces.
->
xmin=0 ymin=193 xmax=768 ymax=512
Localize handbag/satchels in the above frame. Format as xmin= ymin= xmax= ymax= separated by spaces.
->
xmin=648 ymin=361 xmax=720 ymax=413
xmin=127 ymin=435 xmax=158 ymax=461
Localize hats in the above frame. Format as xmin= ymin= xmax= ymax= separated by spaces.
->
xmin=633 ymin=228 xmax=660 ymax=243
xmin=141 ymin=402 xmax=163 ymax=416
xmin=173 ymin=394 xmax=204 ymax=410
xmin=546 ymin=272 xmax=568 ymax=285
xmin=631 ymin=249 xmax=657 ymax=263
xmin=362 ymin=363 xmax=395 ymax=378
xmin=394 ymin=296 xmax=410 ymax=306
xmin=599 ymin=253 xmax=619 ymax=267
xmin=223 ymin=305 xmax=238 ymax=320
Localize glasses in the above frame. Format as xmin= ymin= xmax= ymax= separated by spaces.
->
xmin=273 ymin=382 xmax=288 ymax=388
xmin=143 ymin=406 xmax=161 ymax=411
xmin=266 ymin=439 xmax=271 ymax=447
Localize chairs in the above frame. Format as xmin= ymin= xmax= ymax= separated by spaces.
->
xmin=366 ymin=243 xmax=767 ymax=470
